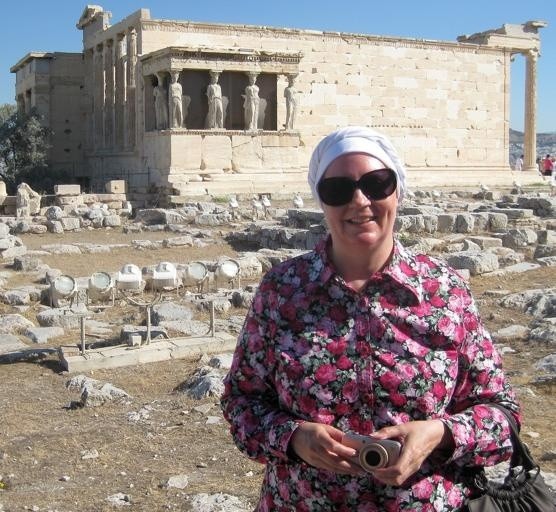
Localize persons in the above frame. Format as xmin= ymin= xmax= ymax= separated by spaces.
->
xmin=220 ymin=122 xmax=523 ymax=510
xmin=543 ymin=154 xmax=554 ymax=176
xmin=169 ymin=72 xmax=184 ymax=128
xmin=204 ymin=72 xmax=223 ymax=128
xmin=242 ymin=75 xmax=261 ymax=130
xmin=153 ymin=76 xmax=168 ymax=129
xmin=514 ymin=154 xmax=524 ymax=171
xmin=282 ymin=76 xmax=304 ymax=131
xmin=536 ymin=155 xmax=544 ymax=173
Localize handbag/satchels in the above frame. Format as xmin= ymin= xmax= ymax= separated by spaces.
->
xmin=466 ymin=404 xmax=554 ymax=512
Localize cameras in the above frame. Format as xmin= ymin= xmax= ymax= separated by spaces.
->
xmin=340 ymin=431 xmax=402 ymax=476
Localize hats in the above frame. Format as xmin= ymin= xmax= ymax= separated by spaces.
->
xmin=309 ymin=127 xmax=407 ymax=209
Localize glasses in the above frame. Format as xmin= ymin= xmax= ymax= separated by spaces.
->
xmin=317 ymin=168 xmax=399 ymax=206
